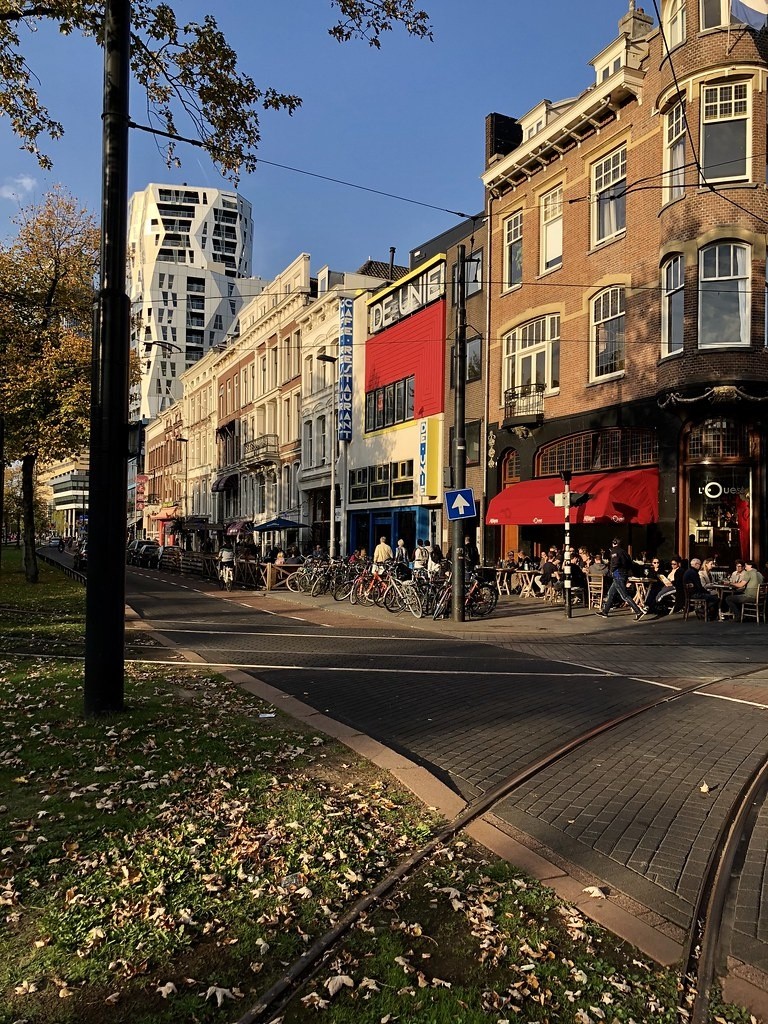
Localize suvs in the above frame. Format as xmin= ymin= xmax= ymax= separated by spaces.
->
xmin=126 ymin=539 xmax=160 ymax=566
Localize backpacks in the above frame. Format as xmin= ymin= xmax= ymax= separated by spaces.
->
xmin=468 ymin=543 xmax=478 ymax=562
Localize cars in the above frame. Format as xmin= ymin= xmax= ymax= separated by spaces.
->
xmin=74 ymin=542 xmax=88 ymax=568
xmin=138 ymin=545 xmax=161 ymax=569
xmin=50 ymin=537 xmax=61 ymax=548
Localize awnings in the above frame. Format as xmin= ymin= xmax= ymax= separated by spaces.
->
xmin=149 ymin=507 xmax=177 ymax=519
xmin=127 ymin=516 xmax=142 ymax=528
xmin=485 ymin=468 xmax=659 ymax=525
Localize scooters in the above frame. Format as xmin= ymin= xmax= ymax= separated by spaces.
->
xmin=59 ymin=544 xmax=65 ymax=554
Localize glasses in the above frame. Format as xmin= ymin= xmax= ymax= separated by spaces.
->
xmin=653 ymin=562 xmax=659 ymax=564
xmin=671 ymin=564 xmax=676 ymax=565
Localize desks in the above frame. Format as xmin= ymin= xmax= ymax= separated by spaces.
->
xmin=515 ymin=570 xmax=539 ymax=598
xmin=629 ymin=577 xmax=658 ymax=613
xmin=493 ymin=569 xmax=514 ymax=596
xmin=708 ymin=584 xmax=739 ymax=621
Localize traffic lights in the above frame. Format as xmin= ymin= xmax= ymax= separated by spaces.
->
xmin=570 ymin=491 xmax=590 ymax=507
xmin=549 ymin=493 xmax=566 ymax=509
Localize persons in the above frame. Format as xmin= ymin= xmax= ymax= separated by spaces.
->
xmin=411 ymin=539 xmax=429 ymax=561
xmin=698 ymin=558 xmax=718 ymax=595
xmin=218 ymin=538 xmax=235 ymax=580
xmin=464 ymin=537 xmax=480 ymax=581
xmin=201 ymin=537 xmax=214 ymax=552
xmin=595 ymin=537 xmax=644 ymax=622
xmin=682 ymin=558 xmax=720 ymax=620
xmin=631 ymin=553 xmax=684 ymax=615
xmin=588 ymin=555 xmax=610 ymax=599
xmin=724 ymin=559 xmax=746 ymax=584
xmin=350 ymin=536 xmax=393 ymax=563
xmin=72 ymin=538 xmax=87 ymax=571
xmin=428 ymin=545 xmax=443 ymax=578
xmin=506 ymin=545 xmax=609 ymax=605
xmin=395 ymin=539 xmax=409 ymax=562
xmin=424 ymin=540 xmax=433 ymax=552
xmin=58 ymin=536 xmax=72 ymax=551
xmin=236 ymin=534 xmax=304 ymax=578
xmin=723 ymin=560 xmax=764 ymax=621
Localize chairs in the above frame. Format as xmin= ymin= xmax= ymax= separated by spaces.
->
xmin=682 ymin=584 xmax=707 ymax=622
xmin=739 ymin=583 xmax=767 ymax=625
xmin=540 ymin=572 xmax=622 ymax=611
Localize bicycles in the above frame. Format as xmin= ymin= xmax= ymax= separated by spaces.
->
xmin=215 ymin=555 xmax=234 ymax=592
xmin=286 ymin=553 xmax=499 ymax=624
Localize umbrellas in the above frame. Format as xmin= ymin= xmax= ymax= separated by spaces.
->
xmin=251 ymin=516 xmax=310 ymax=543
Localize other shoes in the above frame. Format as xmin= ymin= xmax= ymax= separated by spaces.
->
xmin=732 ymin=611 xmax=745 ymax=622
xmin=572 ymin=597 xmax=581 ymax=605
xmin=595 ymin=612 xmax=608 ymax=618
xmin=695 ymin=609 xmax=705 ymax=620
xmin=620 ymin=601 xmax=628 ymax=608
xmin=594 ymin=604 xmax=602 ymax=608
xmin=633 ymin=612 xmax=644 ymax=622
xmin=535 ymin=593 xmax=545 ymax=599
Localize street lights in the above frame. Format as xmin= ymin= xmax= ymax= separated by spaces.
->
xmin=316 ymin=352 xmax=337 ymax=562
xmin=131 ymin=463 xmax=138 ymax=540
xmin=176 ymin=437 xmax=187 ymax=524
xmin=559 ymin=468 xmax=575 ymax=619
